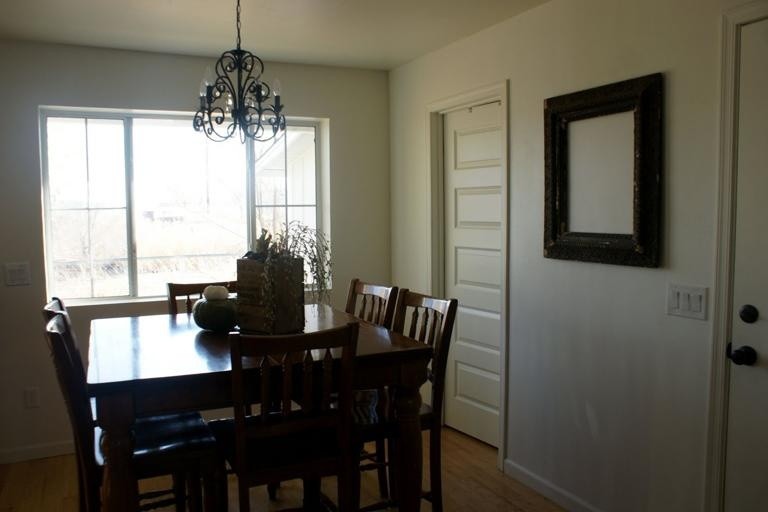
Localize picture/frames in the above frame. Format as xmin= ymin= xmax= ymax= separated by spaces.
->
xmin=542 ymin=72 xmax=665 ymax=269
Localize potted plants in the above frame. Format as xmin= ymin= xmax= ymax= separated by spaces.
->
xmin=237 ymin=219 xmax=336 ymax=332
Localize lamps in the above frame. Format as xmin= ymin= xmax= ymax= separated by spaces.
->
xmin=190 ymin=0 xmax=285 ymax=143
